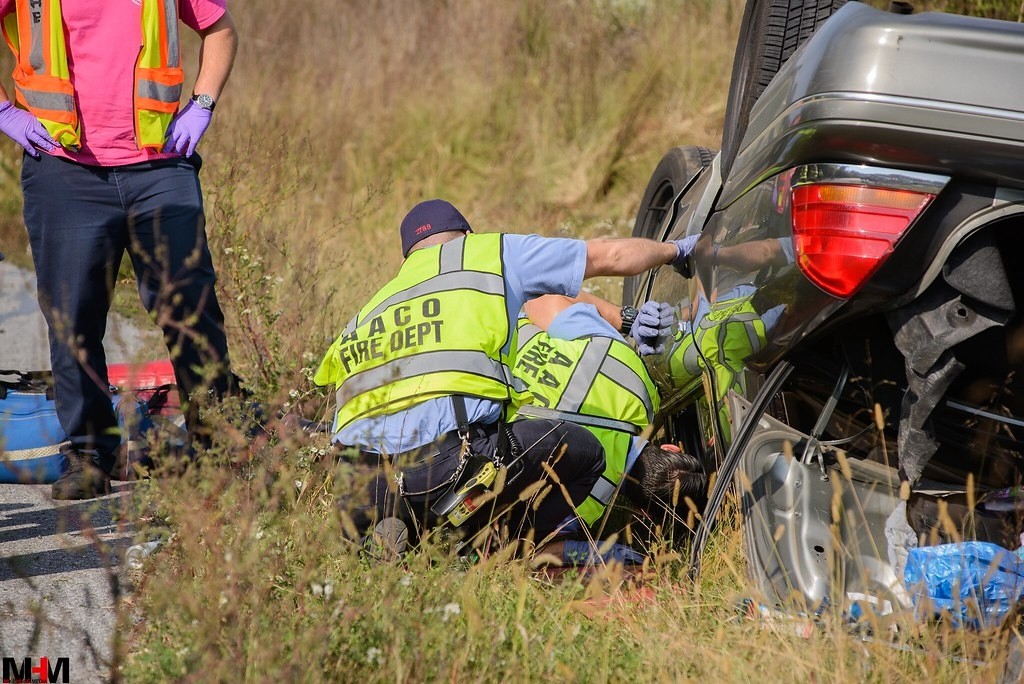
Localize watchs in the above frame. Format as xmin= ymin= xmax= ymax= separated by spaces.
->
xmin=621 ymin=304 xmax=639 ymax=334
xmin=193 ymin=94 xmax=216 ymax=112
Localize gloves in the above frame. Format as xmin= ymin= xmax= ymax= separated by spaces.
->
xmin=668 ymin=234 xmax=700 ymax=279
xmin=661 ymin=302 xmax=682 ymax=354
xmin=162 ymin=99 xmax=213 ymax=158
xmin=562 ymin=539 xmax=644 ymax=565
xmin=629 ymin=300 xmax=661 ymax=355
xmin=0 ymin=101 xmax=61 ymax=157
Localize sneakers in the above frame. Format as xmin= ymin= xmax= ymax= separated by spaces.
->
xmin=51 ymin=445 xmax=121 ymax=500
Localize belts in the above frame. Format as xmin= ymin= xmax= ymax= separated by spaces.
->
xmin=358 ymin=425 xmax=481 ymax=465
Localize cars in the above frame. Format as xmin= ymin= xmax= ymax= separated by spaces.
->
xmin=619 ymin=1 xmax=1024 ymax=626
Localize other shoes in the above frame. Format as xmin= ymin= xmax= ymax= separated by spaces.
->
xmin=365 ymin=517 xmax=408 ymax=567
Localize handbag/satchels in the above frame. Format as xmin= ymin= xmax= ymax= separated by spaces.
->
xmin=0 ymin=370 xmax=150 ymax=483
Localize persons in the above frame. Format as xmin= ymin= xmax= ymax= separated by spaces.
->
xmin=0 ymin=1 xmax=254 ymax=500
xmin=508 ymin=289 xmax=706 ymax=573
xmin=315 ymin=199 xmax=702 ymax=569
xmin=656 ymin=231 xmax=798 ymax=459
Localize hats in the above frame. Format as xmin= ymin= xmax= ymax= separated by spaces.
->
xmin=400 ymin=199 xmax=474 ymax=259
